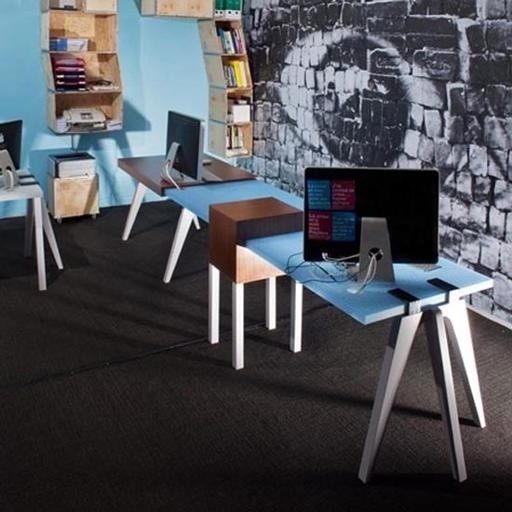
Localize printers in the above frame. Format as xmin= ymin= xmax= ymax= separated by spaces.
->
xmin=63 ymin=106 xmax=108 ymax=134
xmin=48 ymin=151 xmax=98 ymax=178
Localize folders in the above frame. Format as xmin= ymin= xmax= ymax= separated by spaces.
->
xmin=215 ymin=0 xmax=224 ymax=17
xmin=234 ymin=0 xmax=241 ymax=20
xmin=224 ymin=0 xmax=233 ymax=18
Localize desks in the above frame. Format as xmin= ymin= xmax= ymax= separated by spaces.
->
xmin=170 ymin=180 xmax=495 ymax=487
xmin=2 ymin=168 xmax=63 ymax=292
xmin=118 ymin=153 xmax=254 ymax=242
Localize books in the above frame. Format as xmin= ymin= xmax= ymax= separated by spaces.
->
xmin=218 ymin=27 xmax=244 ymax=54
xmin=223 ymin=60 xmax=248 ymax=88
xmin=226 ymin=125 xmax=249 ymax=156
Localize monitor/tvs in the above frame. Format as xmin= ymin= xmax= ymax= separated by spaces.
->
xmin=160 ymin=110 xmax=206 ymax=183
xmin=302 ymin=166 xmax=440 ymax=296
xmin=0 ymin=119 xmax=23 ymax=191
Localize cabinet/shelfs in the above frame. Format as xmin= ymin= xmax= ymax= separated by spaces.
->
xmin=139 ymin=1 xmax=244 ymax=18
xmin=197 ymin=19 xmax=254 ymax=158
xmin=41 ymin=1 xmax=124 ymax=133
